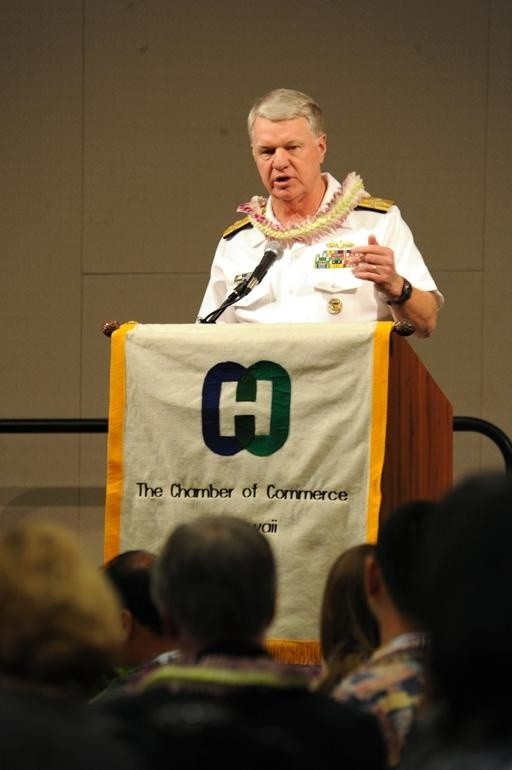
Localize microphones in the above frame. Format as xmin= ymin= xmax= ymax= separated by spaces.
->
xmin=243 ymin=239 xmax=285 ymax=298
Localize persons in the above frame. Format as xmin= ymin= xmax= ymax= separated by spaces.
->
xmin=310 ymin=472 xmax=511 ymax=770
xmin=195 ymin=86 xmax=446 ymax=339
xmin=1 ymin=504 xmax=391 ymax=770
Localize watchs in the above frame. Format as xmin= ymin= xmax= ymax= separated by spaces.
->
xmin=384 ymin=279 xmax=412 ymax=306
